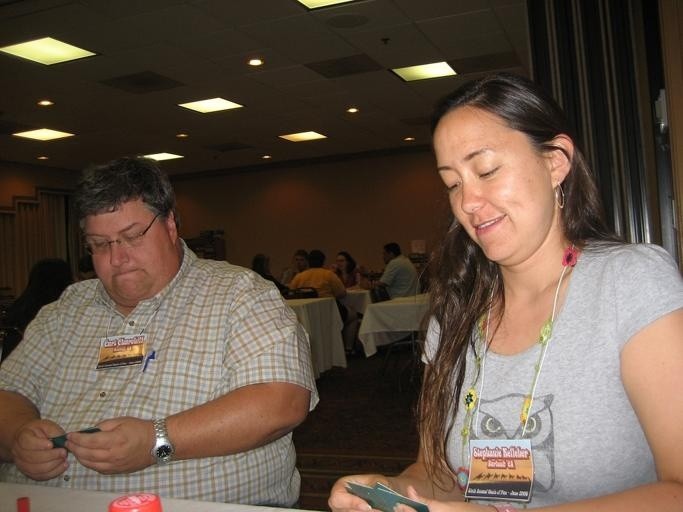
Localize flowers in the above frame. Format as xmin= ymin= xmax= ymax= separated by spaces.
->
xmin=560 ymin=246 xmax=579 ymax=269
xmin=463 ymin=390 xmax=475 ymax=410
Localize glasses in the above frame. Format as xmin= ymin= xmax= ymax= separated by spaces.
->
xmin=84 ymin=211 xmax=164 ymax=257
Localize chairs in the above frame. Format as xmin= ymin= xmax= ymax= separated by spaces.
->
xmin=0 ymin=325 xmax=25 ymax=367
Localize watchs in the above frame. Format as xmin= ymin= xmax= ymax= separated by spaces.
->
xmin=151 ymin=418 xmax=174 ymax=463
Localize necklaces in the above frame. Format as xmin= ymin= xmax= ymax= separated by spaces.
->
xmin=456 ymin=241 xmax=577 ymax=509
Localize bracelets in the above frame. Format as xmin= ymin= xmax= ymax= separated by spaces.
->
xmin=490 ymin=503 xmax=517 ymax=512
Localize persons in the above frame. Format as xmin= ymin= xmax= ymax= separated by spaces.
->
xmin=328 ymin=71 xmax=683 ymax=512
xmin=0 ymin=158 xmax=320 ymax=507
